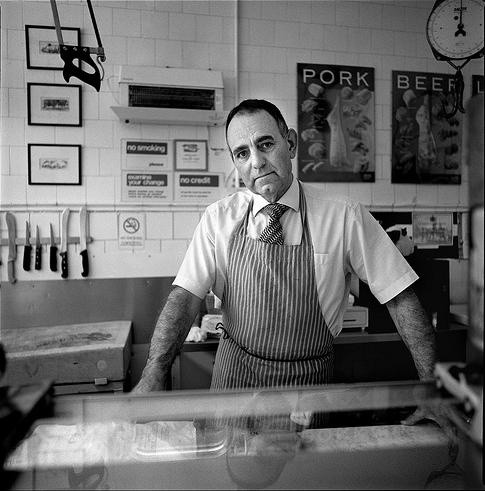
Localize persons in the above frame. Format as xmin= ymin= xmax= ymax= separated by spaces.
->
xmin=127 ymin=99 xmax=471 ymax=445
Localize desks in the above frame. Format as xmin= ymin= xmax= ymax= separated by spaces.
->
xmin=170 ymin=324 xmax=468 ymax=388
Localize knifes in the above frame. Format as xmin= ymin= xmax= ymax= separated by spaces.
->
xmin=3 ymin=212 xmax=20 ymax=287
xmin=59 ymin=204 xmax=70 ymax=279
xmin=45 ymin=222 xmax=60 ymax=272
xmin=78 ymin=207 xmax=91 ymax=281
xmin=33 ymin=225 xmax=43 ymax=271
xmin=22 ymin=219 xmax=33 ymax=274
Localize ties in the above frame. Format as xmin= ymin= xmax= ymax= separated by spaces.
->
xmin=259 ymin=204 xmax=290 ymax=245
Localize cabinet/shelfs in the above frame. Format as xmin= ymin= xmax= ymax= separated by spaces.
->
xmin=2 ymin=321 xmax=134 ymax=393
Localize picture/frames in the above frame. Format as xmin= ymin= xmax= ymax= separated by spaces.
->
xmin=25 ymin=24 xmax=81 ymax=70
xmin=26 ymin=82 xmax=82 ymax=127
xmin=27 ymin=143 xmax=83 ymax=185
